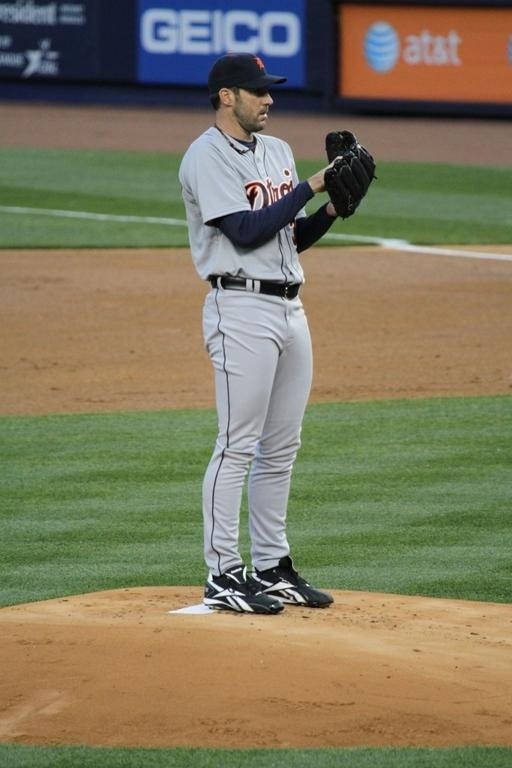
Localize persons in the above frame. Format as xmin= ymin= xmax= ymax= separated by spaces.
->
xmin=177 ymin=50 xmax=383 ymax=614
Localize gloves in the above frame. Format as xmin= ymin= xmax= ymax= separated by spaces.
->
xmin=210 ymin=274 xmax=299 ymax=301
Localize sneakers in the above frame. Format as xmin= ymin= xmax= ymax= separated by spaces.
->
xmin=202 ymin=562 xmax=283 ymax=614
xmin=250 ymin=554 xmax=335 ymax=609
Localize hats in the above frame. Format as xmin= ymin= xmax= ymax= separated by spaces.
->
xmin=207 ymin=50 xmax=286 ymax=93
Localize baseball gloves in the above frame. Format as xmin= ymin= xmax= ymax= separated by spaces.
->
xmin=324 ymin=131 xmax=377 ymax=220
